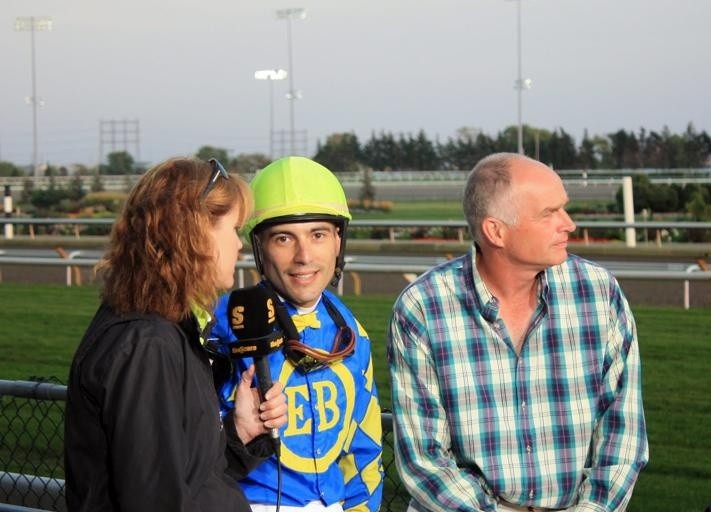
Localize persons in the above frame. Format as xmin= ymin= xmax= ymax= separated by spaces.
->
xmin=199 ymin=155 xmax=388 ymax=511
xmin=61 ymin=154 xmax=288 ymax=512
xmin=386 ymin=151 xmax=655 ymax=510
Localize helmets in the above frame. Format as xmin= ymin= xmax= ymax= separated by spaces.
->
xmin=243 ymin=155 xmax=352 ymax=250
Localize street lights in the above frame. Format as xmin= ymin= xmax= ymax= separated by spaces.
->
xmin=14 ymin=15 xmax=53 ymax=174
xmin=254 ymin=7 xmax=308 ymax=153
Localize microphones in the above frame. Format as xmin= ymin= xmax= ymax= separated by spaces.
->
xmin=228 ymin=286 xmax=285 ymax=457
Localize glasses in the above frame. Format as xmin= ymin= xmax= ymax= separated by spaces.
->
xmin=201 ymin=157 xmax=229 ymax=201
